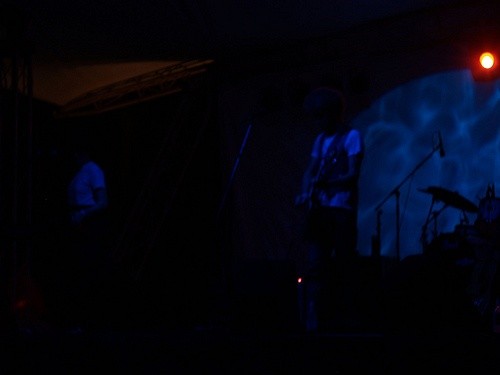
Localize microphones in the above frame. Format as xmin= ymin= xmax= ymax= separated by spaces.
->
xmin=439 ymin=133 xmax=445 ymax=159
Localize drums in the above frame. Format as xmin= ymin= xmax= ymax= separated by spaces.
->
xmin=433 ymin=234 xmax=497 ymax=298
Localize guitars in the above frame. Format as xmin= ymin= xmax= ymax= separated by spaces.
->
xmin=290 ymin=149 xmax=339 ymax=238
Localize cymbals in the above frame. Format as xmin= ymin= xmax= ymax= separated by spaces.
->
xmin=427 ymin=187 xmax=479 ymax=214
